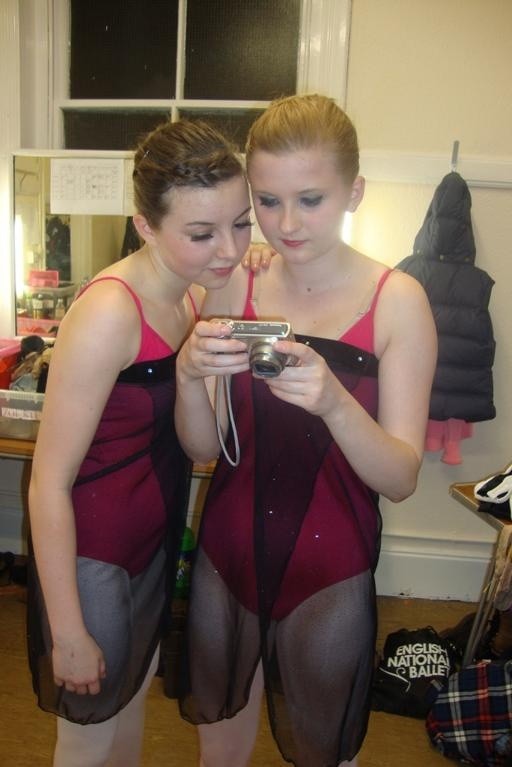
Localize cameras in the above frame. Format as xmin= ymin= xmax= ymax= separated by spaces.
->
xmin=209 ymin=317 xmax=297 ymax=380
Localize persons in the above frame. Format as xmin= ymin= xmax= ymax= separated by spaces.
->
xmin=173 ymin=94 xmax=443 ymax=766
xmin=23 ymin=116 xmax=279 ymax=766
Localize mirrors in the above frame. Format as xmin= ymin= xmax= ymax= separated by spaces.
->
xmin=7 ymin=147 xmax=146 ymax=346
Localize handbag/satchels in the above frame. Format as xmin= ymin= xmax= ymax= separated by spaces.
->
xmin=373 ymin=625 xmax=451 ymax=715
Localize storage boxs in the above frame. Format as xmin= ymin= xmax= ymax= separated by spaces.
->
xmin=0 ymin=388 xmax=44 ymax=441
xmin=23 ymin=281 xmax=78 ymax=319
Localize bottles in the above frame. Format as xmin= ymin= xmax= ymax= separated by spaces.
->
xmin=172 ymin=527 xmax=196 ymax=599
xmin=20 ymin=291 xmax=67 ymax=322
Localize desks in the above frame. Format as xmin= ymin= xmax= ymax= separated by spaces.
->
xmin=450 ymin=481 xmax=511 ymax=671
xmin=1 ymin=438 xmax=216 ymax=478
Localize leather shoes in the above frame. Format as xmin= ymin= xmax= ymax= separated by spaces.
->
xmin=440 ymin=612 xmax=479 ymax=665
